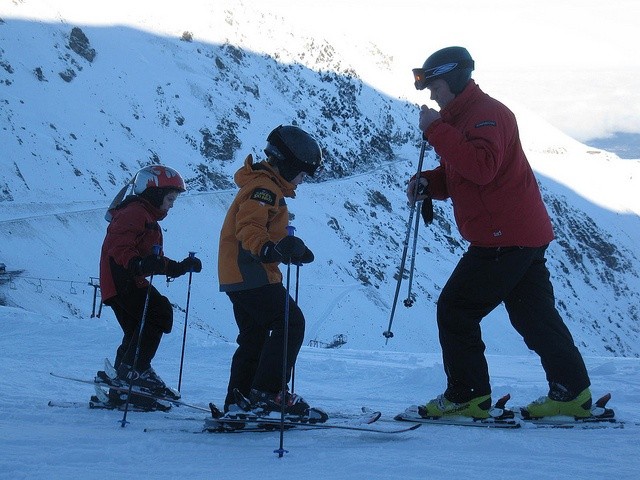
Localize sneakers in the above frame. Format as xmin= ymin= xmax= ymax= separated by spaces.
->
xmin=116 ymin=362 xmax=180 ymax=398
xmin=526 ymin=387 xmax=591 ymax=417
xmin=248 ymin=388 xmax=327 ymax=422
xmin=109 ymin=386 xmax=171 ymax=411
xmin=426 ymin=393 xmax=491 ymax=418
xmin=223 ymin=390 xmax=253 ymax=421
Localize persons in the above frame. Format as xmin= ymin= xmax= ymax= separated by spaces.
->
xmin=215 ymin=125 xmax=320 ymax=428
xmin=91 ymin=165 xmax=201 ymax=413
xmin=407 ymin=46 xmax=592 ymax=420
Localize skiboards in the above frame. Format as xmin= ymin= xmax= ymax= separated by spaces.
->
xmin=48 ymin=363 xmax=211 ymax=413
xmin=144 ymin=392 xmax=423 ymax=433
xmin=362 ymin=393 xmax=640 ymax=429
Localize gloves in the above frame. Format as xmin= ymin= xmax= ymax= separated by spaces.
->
xmin=180 ymin=256 xmax=202 ymax=274
xmin=145 ymin=254 xmax=180 ymax=278
xmin=302 ymin=245 xmax=313 ymax=262
xmin=261 ymin=235 xmax=305 ymax=264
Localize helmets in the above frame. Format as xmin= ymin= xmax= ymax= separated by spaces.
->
xmin=264 ymin=124 xmax=321 ymax=182
xmin=412 ymin=46 xmax=473 ymax=94
xmin=133 ymin=165 xmax=185 ymax=193
